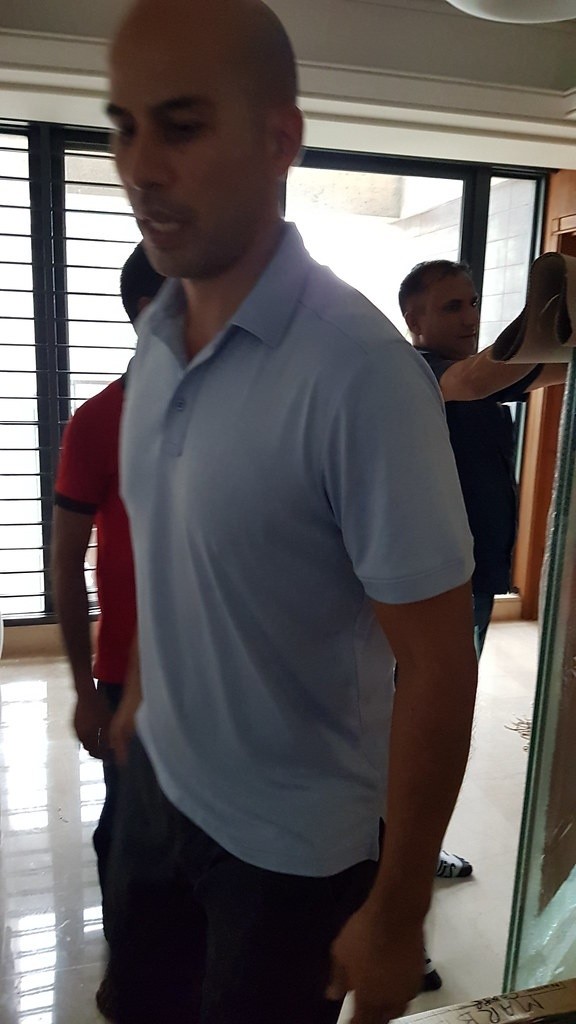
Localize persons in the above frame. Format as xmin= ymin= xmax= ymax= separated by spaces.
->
xmin=106 ymin=0 xmax=479 ymax=1024
xmin=49 ymin=237 xmax=167 ymax=1024
xmin=392 ymin=259 xmax=569 ymax=992
